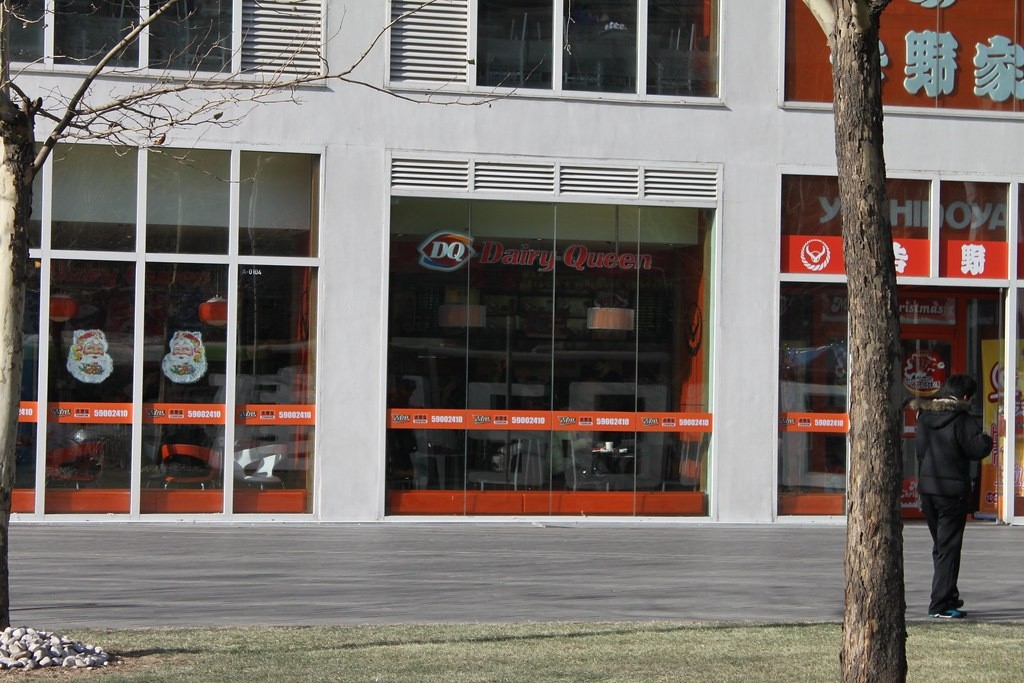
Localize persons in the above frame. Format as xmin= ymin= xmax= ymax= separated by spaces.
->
xmin=387 ymin=378 xmax=419 ymax=489
xmin=916 ymin=371 xmax=994 ymax=619
xmin=547 ymin=395 xmax=596 ymax=492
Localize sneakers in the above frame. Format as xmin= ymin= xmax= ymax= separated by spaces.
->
xmin=928 ymin=608 xmax=967 ymax=618
xmin=951 ymin=598 xmax=964 ymax=609
